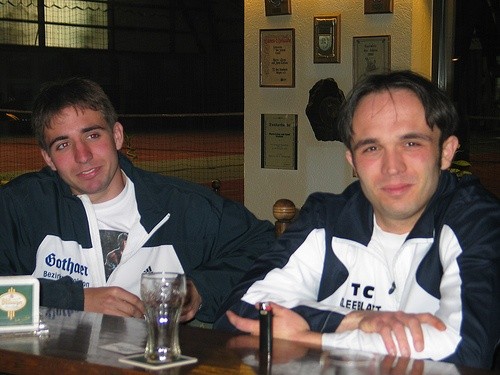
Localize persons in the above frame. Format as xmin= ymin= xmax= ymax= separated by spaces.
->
xmin=0 ymin=72 xmax=276 ymax=331
xmin=105 ymin=233 xmax=127 ymax=279
xmin=0 ymin=307 xmax=149 ymax=368
xmin=214 ymin=71 xmax=500 ymax=368
xmin=226 ymin=334 xmax=459 ymax=375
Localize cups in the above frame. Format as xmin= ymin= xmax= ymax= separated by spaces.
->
xmin=140 ymin=271 xmax=185 ymax=364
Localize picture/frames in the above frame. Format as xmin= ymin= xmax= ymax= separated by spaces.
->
xmin=313 ymin=14 xmax=341 ymax=64
xmin=364 ymin=0 xmax=394 ymax=14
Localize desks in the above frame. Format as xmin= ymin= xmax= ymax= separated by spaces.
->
xmin=0 ymin=306 xmax=500 ymax=375
